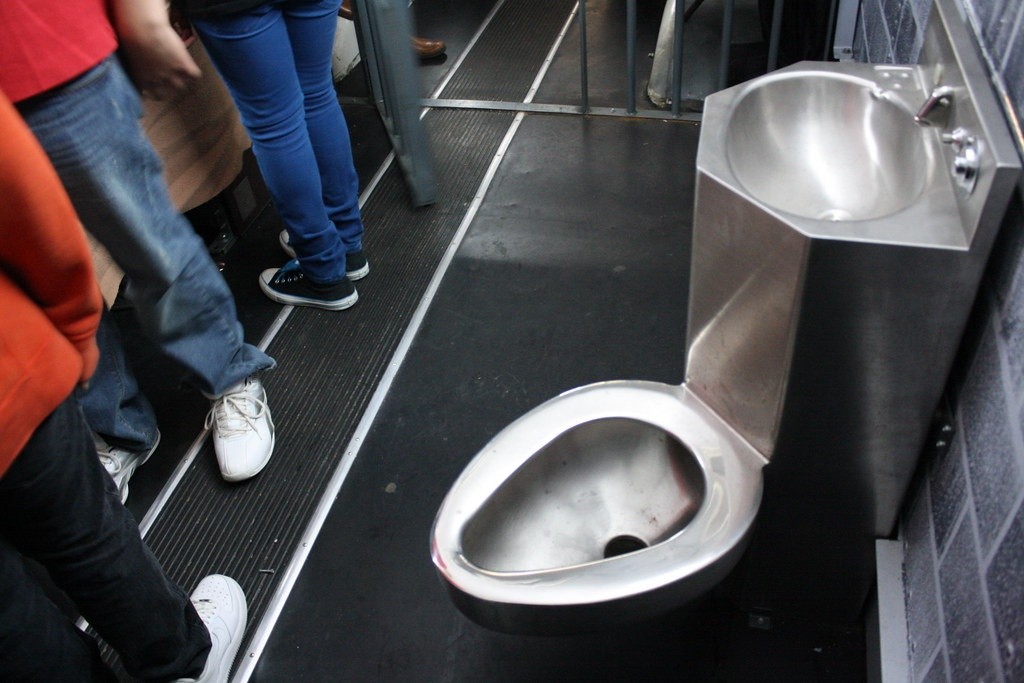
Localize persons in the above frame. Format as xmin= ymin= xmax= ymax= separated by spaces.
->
xmin=185 ymin=0 xmax=369 ymax=310
xmin=0 ymin=0 xmax=278 ymax=504
xmin=0 ymin=89 xmax=249 ymax=682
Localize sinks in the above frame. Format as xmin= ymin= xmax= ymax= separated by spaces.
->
xmin=720 ymin=65 xmax=933 ymax=225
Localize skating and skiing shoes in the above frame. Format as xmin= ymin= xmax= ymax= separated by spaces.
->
xmin=258 ymin=258 xmax=359 ymax=311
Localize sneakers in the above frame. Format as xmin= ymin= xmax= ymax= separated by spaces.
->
xmin=95 ymin=426 xmax=161 ymax=506
xmin=171 ymin=574 xmax=247 ymax=683
xmin=200 ymin=372 xmax=275 ymax=482
xmin=279 ymin=228 xmax=370 ymax=282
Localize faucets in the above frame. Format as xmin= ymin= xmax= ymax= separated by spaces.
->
xmin=913 ymin=83 xmax=956 ymax=127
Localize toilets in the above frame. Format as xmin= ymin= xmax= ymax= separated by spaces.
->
xmin=427 ymin=374 xmax=779 ymax=642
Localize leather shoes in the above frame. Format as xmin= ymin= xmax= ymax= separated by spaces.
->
xmin=411 ymin=34 xmax=446 ymax=60
